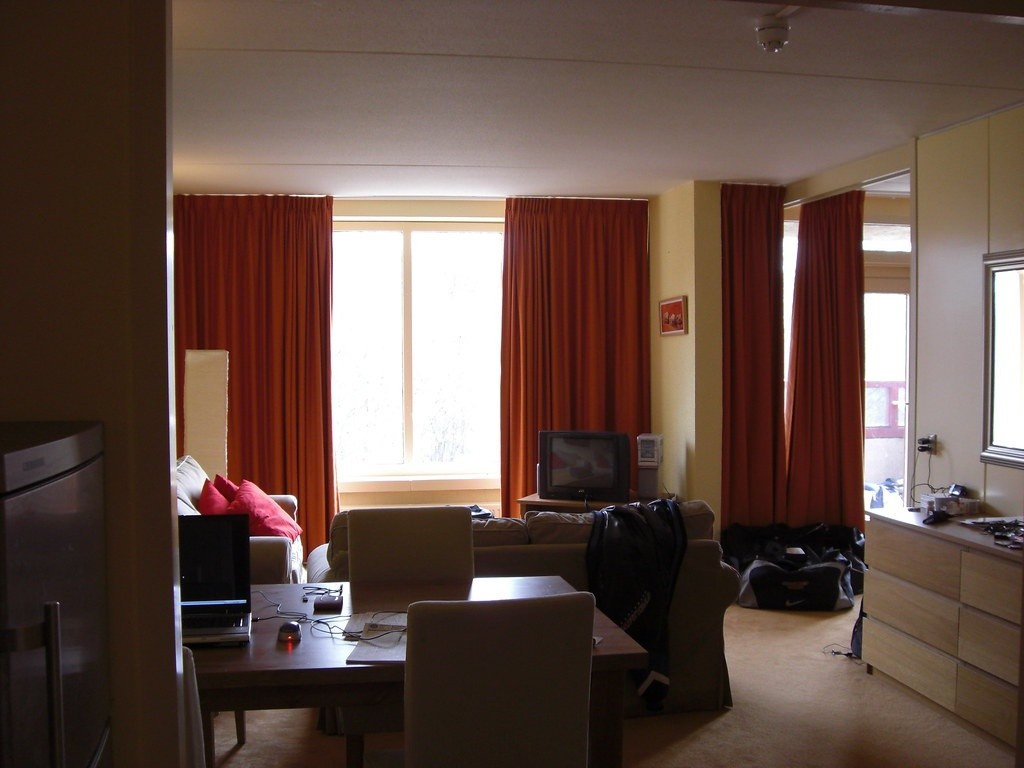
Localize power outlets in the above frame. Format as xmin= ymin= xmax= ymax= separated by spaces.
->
xmin=927 ymin=434 xmax=937 ymax=455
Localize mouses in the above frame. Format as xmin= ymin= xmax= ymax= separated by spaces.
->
xmin=278 ymin=621 xmax=302 ymax=641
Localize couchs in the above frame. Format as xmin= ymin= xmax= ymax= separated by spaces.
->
xmin=176 ymin=454 xmax=304 ymax=585
xmin=306 ymin=539 xmax=741 ymax=736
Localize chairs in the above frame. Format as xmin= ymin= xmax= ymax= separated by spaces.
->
xmin=403 ymin=591 xmax=596 ymax=768
xmin=179 ymin=513 xmax=250 ymax=745
xmin=342 ymin=507 xmax=476 ymax=768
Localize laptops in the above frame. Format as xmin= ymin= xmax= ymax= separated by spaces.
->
xmin=177 ymin=514 xmax=252 ymax=645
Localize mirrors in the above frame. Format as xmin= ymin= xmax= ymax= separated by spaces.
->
xmin=980 ymin=248 xmax=1024 ymax=470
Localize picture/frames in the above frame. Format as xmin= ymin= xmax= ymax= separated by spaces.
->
xmin=659 ymin=295 xmax=688 ymax=337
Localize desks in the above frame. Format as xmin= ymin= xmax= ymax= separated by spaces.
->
xmin=185 ymin=575 xmax=650 ymax=768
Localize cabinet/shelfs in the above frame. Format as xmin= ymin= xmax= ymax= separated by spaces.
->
xmin=516 ymin=493 xmax=631 ymax=519
xmin=864 ymin=506 xmax=1024 ymax=750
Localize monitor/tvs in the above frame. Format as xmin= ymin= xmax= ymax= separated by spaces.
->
xmin=536 ymin=431 xmax=631 ymax=504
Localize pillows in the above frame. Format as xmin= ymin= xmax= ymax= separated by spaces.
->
xmin=326 ymin=510 xmax=529 ymax=573
xmin=197 ymin=478 xmax=231 ymax=515
xmin=523 ymin=499 xmax=716 ymax=545
xmin=213 ymin=472 xmax=239 ymax=503
xmin=228 ymin=479 xmax=304 ymax=544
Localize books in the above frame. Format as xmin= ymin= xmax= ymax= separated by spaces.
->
xmin=346 ymin=622 xmax=406 ymax=665
